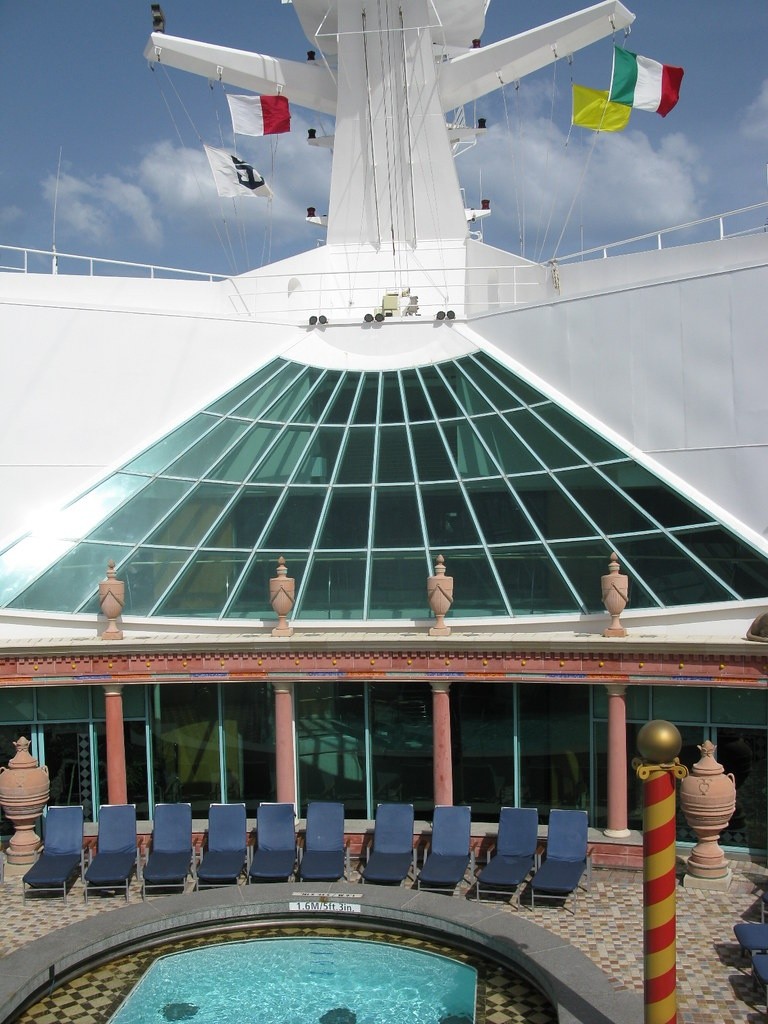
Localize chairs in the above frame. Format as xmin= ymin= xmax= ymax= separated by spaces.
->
xmin=22 ymin=802 xmax=350 ymax=904
xmin=476 ymin=807 xmax=592 ymax=915
xmin=734 ymin=892 xmax=768 ymax=1016
xmin=361 ymin=803 xmax=418 ymax=887
xmin=417 ymin=805 xmax=475 ymax=892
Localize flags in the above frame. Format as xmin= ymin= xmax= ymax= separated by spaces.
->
xmin=573 ymin=84 xmax=632 ymax=131
xmin=609 ymin=45 xmax=684 ymax=117
xmin=204 ymin=143 xmax=273 ymax=198
xmin=226 ymin=93 xmax=291 ymax=136
